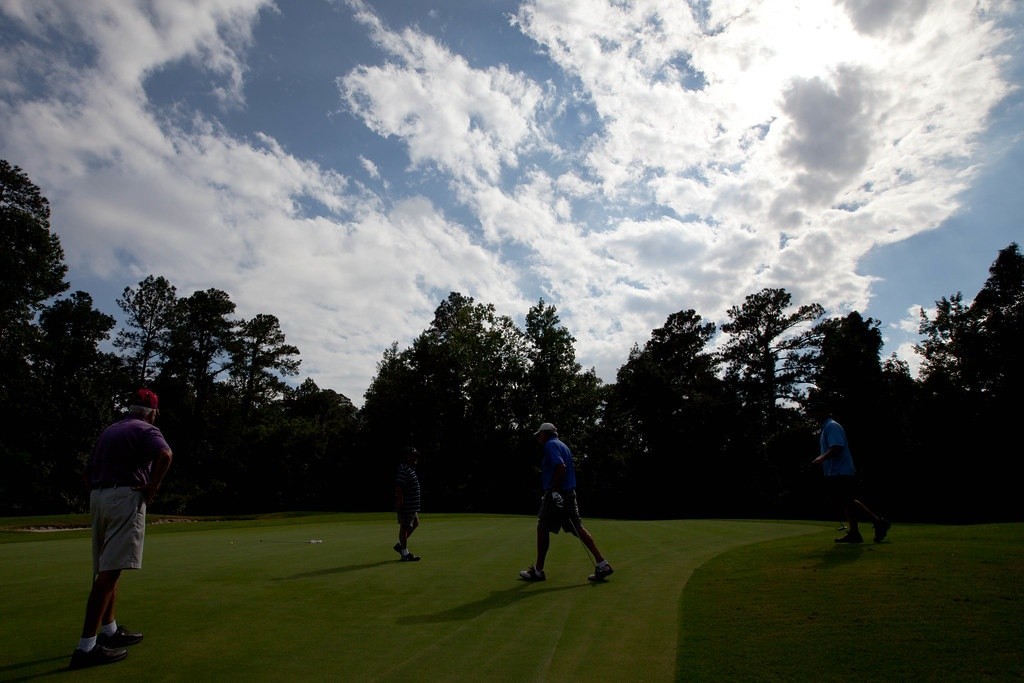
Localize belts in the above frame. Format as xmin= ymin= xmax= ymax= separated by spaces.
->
xmin=91 ymin=480 xmax=140 ymax=490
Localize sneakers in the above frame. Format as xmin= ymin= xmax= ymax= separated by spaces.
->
xmin=589 ymin=564 xmax=613 ymax=581
xmin=96 ymin=626 xmax=143 ymax=649
xmin=520 ymin=566 xmax=545 ymax=581
xmin=70 ymin=638 xmax=128 ymax=668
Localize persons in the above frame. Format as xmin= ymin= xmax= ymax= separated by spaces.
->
xmin=70 ymin=389 xmax=172 ymax=668
xmin=807 ymin=399 xmax=891 ymax=543
xmin=394 ymin=447 xmax=421 ymax=560
xmin=520 ymin=422 xmax=613 ymax=581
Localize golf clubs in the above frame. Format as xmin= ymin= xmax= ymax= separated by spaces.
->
xmin=556 ymin=494 xmax=597 ymax=568
xmin=260 ymin=539 xmax=322 ymax=544
xmin=837 ymin=516 xmax=848 ymax=531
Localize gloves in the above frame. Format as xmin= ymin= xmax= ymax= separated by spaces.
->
xmin=551 ymin=490 xmax=564 ymax=507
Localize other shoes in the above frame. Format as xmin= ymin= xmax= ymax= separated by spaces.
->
xmin=401 ymin=552 xmax=421 ymax=561
xmin=393 ymin=543 xmax=402 ymax=555
xmin=872 ymin=518 xmax=891 ymax=542
xmin=835 ymin=531 xmax=863 ymax=543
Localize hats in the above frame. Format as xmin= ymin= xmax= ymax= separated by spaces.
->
xmin=131 ymin=388 xmax=161 ymax=415
xmin=534 ymin=423 xmax=557 ymax=436
xmin=403 ymin=446 xmax=422 ymax=455
xmin=806 ymin=401 xmax=827 ymax=413
